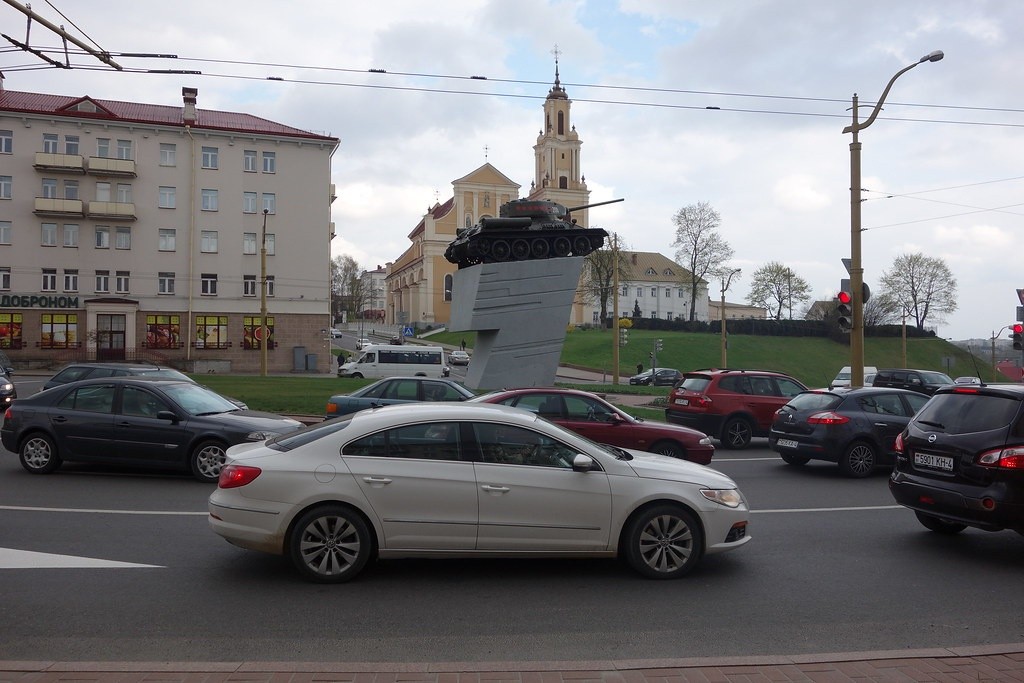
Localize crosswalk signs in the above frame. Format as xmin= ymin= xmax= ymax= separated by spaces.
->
xmin=404 ymin=328 xmax=414 ymax=337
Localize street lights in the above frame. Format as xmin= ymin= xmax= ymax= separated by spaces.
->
xmin=901 ymin=300 xmax=929 ymax=368
xmin=720 ymin=268 xmax=741 ymax=369
xmin=261 ymin=208 xmax=270 ymax=375
xmin=991 ymin=324 xmax=1014 ymax=382
xmin=842 ymin=50 xmax=945 ymax=388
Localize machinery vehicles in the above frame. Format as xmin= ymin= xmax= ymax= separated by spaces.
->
xmin=443 ymin=197 xmax=625 ymax=268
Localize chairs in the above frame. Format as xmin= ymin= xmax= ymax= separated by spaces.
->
xmin=756 ymin=381 xmax=770 ymax=396
xmin=879 ymin=401 xmax=899 ymax=415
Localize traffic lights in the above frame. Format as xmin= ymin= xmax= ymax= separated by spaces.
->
xmin=1013 ymin=323 xmax=1024 ymax=349
xmin=838 ymin=290 xmax=853 ymax=329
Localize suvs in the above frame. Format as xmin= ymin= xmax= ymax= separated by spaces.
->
xmin=889 ymin=383 xmax=1024 ymax=538
xmin=665 ymin=367 xmax=810 ymax=450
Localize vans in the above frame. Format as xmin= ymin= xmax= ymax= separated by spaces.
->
xmin=336 ymin=343 xmax=444 ymax=380
xmin=871 ymin=367 xmax=955 ymax=397
xmin=832 ymin=366 xmax=877 ymax=387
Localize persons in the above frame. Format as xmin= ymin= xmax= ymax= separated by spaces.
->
xmin=636 ymin=362 xmax=643 ymax=374
xmin=462 ymin=339 xmax=466 ymax=350
xmin=346 ymin=355 xmax=352 ymax=363
xmin=479 ymin=422 xmax=531 ymax=464
xmin=434 ymin=385 xmax=447 ymax=401
xmin=337 ymin=353 xmax=345 ymax=366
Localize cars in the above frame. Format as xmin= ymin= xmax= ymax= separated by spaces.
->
xmin=431 ymin=385 xmax=715 ymax=465
xmin=356 ymin=339 xmax=372 ymax=350
xmin=768 ymin=385 xmax=935 ymax=479
xmin=953 ymin=376 xmax=981 ymax=384
xmin=389 ymin=336 xmax=402 ymax=345
xmin=443 ymin=360 xmax=450 ymax=377
xmin=1 ymin=375 xmax=309 ymax=484
xmin=208 ymin=400 xmax=754 ymax=586
xmin=0 ymin=365 xmax=18 ymax=413
xmin=42 ymin=361 xmax=249 ymax=412
xmin=448 ymin=350 xmax=470 ymax=365
xmin=330 ymin=327 xmax=342 ymax=339
xmin=323 ymin=375 xmax=541 ymax=420
xmin=630 ymin=367 xmax=684 ymax=388
